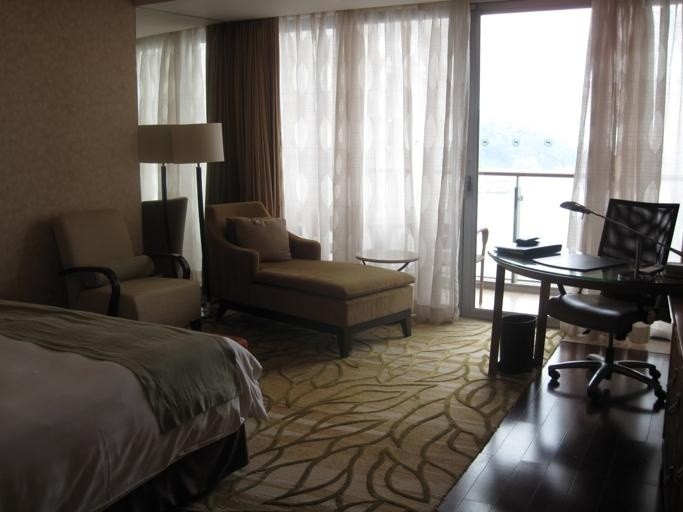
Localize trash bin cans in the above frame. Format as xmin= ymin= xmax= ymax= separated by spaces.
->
xmin=500 ymin=315 xmax=537 ymax=374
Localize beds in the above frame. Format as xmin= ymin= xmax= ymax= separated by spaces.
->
xmin=1 ymin=302 xmax=264 ymax=511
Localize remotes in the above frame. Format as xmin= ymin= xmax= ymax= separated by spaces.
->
xmin=639 ymin=264 xmax=664 ymax=274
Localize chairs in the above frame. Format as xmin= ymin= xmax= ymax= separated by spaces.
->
xmin=545 ymin=198 xmax=680 ymax=400
xmin=53 ymin=208 xmax=202 ymax=332
xmin=142 ymin=197 xmax=187 ymax=271
xmin=202 ymin=201 xmax=414 ymax=358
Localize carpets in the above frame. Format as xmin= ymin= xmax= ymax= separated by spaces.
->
xmin=181 ymin=302 xmax=566 ymax=512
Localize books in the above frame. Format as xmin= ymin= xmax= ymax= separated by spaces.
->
xmin=495 ymin=244 xmax=562 ymax=259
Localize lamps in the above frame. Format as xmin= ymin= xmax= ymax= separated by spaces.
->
xmin=171 ymin=123 xmax=225 ymax=301
xmin=138 ymin=125 xmax=174 ymax=278
xmin=560 ymin=202 xmax=683 ymax=257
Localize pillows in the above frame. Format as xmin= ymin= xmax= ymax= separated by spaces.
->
xmin=79 ymin=255 xmax=156 ymax=289
xmin=225 ymin=217 xmax=292 ymax=262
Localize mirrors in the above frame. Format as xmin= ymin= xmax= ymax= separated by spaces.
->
xmin=135 ymin=6 xmax=207 ymax=287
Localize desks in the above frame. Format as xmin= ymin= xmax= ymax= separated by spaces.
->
xmin=488 ymin=249 xmax=682 ymax=376
xmin=357 ymin=248 xmax=418 ymax=272
